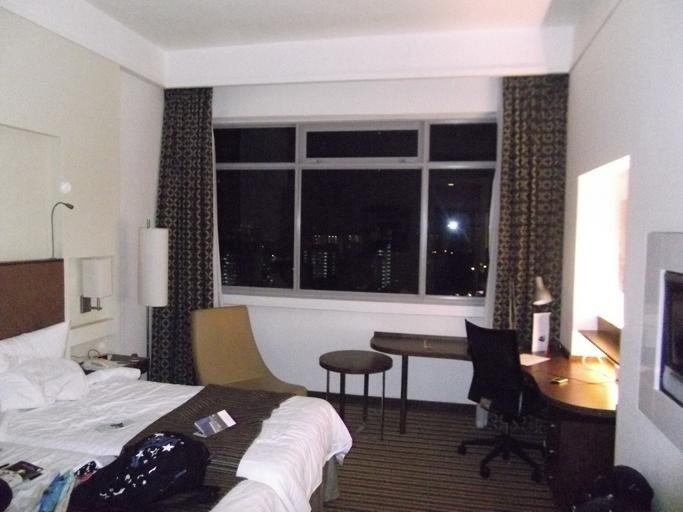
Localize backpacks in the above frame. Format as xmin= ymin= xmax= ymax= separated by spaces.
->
xmin=67 ymin=431 xmax=209 ymax=512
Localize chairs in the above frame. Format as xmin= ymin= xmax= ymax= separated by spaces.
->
xmin=456 ymin=318 xmax=545 ymax=483
xmin=189 ymin=304 xmax=309 ymax=397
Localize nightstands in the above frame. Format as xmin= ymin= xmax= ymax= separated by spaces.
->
xmin=79 ymin=352 xmax=150 ymax=381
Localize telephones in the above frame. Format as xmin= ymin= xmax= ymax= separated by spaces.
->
xmin=82 ymin=358 xmax=115 ymax=371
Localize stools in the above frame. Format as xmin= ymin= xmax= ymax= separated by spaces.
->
xmin=318 ymin=349 xmax=393 ymax=443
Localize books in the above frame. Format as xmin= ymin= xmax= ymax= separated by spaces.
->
xmin=192 ymin=409 xmax=236 ymax=437
xmin=518 ymin=352 xmax=551 ymax=366
xmin=0 ymin=460 xmax=45 ymax=492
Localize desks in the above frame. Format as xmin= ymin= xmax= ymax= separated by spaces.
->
xmin=370 ymin=331 xmax=618 ymax=506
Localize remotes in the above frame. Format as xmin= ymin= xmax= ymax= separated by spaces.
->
xmin=130 ymin=353 xmax=140 ymax=363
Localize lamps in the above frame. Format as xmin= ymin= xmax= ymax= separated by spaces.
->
xmin=138 ymin=217 xmax=169 ymax=381
xmin=507 ymin=274 xmax=552 ymax=332
xmin=79 ymin=258 xmax=113 ymax=314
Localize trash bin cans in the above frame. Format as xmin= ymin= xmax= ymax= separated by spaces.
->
xmin=551 ymin=465 xmax=654 ymax=512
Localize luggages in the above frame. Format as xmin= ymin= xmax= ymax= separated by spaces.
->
xmin=542 ymin=416 xmax=604 ymax=510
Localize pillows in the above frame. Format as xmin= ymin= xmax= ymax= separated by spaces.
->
xmin=0 ymin=320 xmax=72 ymax=370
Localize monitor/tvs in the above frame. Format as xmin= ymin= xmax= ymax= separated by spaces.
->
xmin=660 ymin=270 xmax=683 ymax=406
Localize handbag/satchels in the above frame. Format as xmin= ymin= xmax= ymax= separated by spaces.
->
xmin=573 ymin=466 xmax=653 ymax=512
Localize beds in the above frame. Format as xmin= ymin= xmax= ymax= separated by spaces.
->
xmin=0 ymin=256 xmax=354 ymax=512
